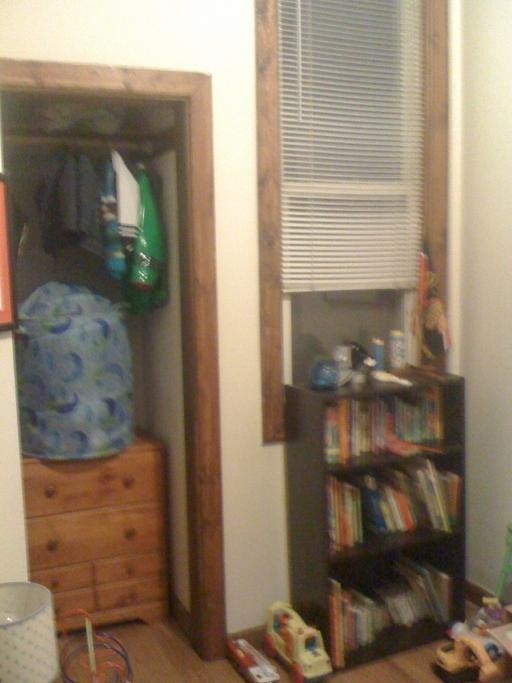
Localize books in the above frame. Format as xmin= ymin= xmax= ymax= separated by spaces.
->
xmin=331 ymin=560 xmax=455 ymax=669
xmin=325 ymin=456 xmax=462 ymax=557
xmin=323 ymin=381 xmax=446 ymax=465
xmin=434 ymin=622 xmax=512 ymax=683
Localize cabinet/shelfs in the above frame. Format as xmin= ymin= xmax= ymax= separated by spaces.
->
xmin=282 ymin=367 xmax=466 ymax=672
xmin=18 ymin=426 xmax=172 ymax=631
